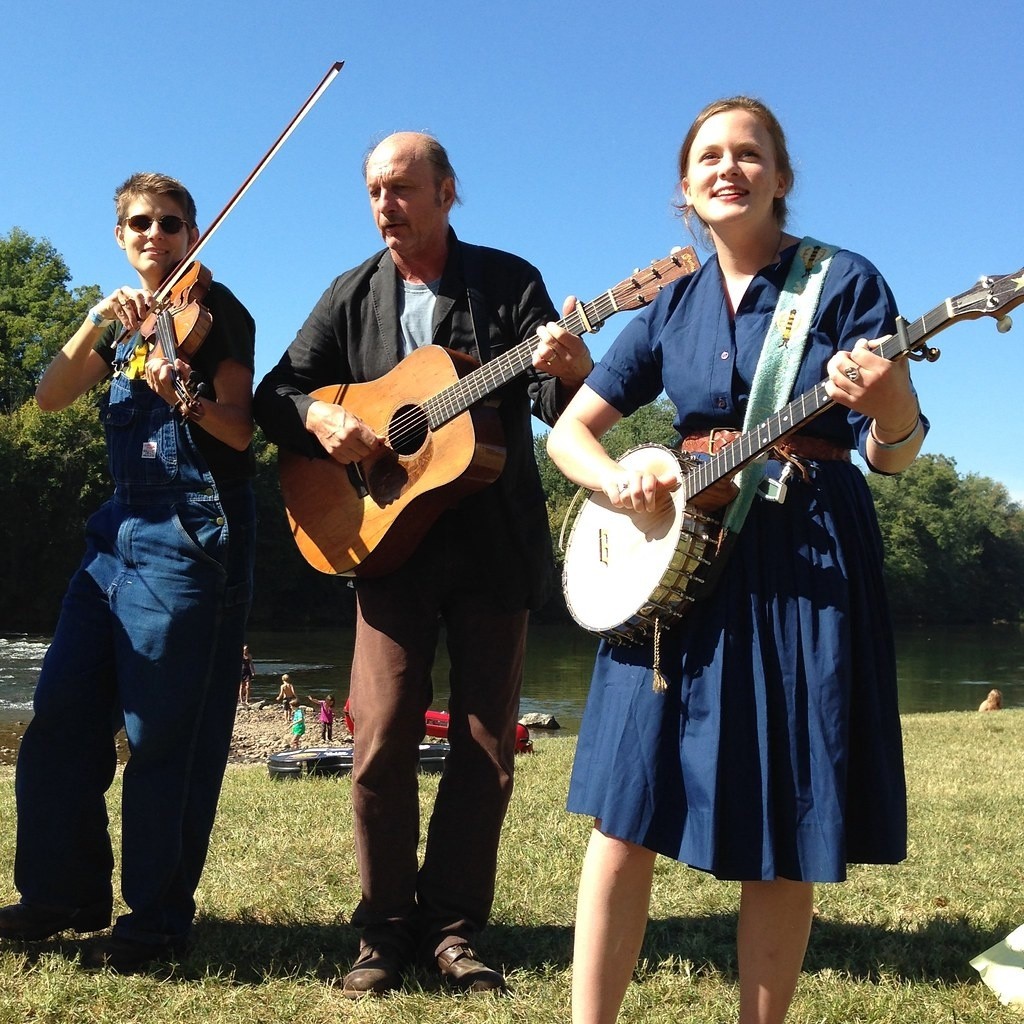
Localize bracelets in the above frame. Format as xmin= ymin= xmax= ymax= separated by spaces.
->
xmin=88 ymin=308 xmax=114 ymax=328
xmin=869 ymin=418 xmax=920 ymax=449
xmin=876 ymin=398 xmax=918 ymax=434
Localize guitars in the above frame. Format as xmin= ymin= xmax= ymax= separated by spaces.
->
xmin=561 ymin=263 xmax=1024 ymax=648
xmin=283 ymin=246 xmax=705 ymax=580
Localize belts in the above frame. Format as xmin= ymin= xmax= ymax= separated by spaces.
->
xmin=678 ymin=429 xmax=855 ymax=463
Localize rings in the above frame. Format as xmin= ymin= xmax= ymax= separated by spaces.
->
xmin=120 ymin=299 xmax=130 ymax=305
xmin=620 ymin=482 xmax=628 ymax=494
xmin=845 ymin=364 xmax=860 ymax=381
xmin=547 ymin=353 xmax=557 ymax=366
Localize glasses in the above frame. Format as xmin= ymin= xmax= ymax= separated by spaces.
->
xmin=123 ymin=215 xmax=189 ymax=235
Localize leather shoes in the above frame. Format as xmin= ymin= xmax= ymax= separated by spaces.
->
xmin=433 ymin=942 xmax=507 ymax=996
xmin=342 ymin=939 xmax=405 ymax=999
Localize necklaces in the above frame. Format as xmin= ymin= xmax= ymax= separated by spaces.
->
xmin=770 ymin=231 xmax=782 ymax=266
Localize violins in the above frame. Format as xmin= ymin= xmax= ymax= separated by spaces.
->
xmin=141 ymin=259 xmax=216 ymax=424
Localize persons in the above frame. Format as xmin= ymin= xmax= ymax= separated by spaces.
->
xmin=254 ymin=131 xmax=594 ymax=998
xmin=343 ymin=698 xmax=354 ymax=738
xmin=308 ymin=693 xmax=336 ymax=744
xmin=289 ymin=699 xmax=305 ymax=749
xmin=239 ymin=645 xmax=255 ymax=706
xmin=276 ymin=674 xmax=297 ymax=725
xmin=978 ymin=689 xmax=1003 ymax=712
xmin=546 ymin=96 xmax=930 ymax=1024
xmin=0 ymin=172 xmax=256 ymax=975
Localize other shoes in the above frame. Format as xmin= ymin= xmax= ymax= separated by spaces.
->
xmin=0 ymin=902 xmax=112 ymax=942
xmin=64 ymin=920 xmax=179 ymax=976
